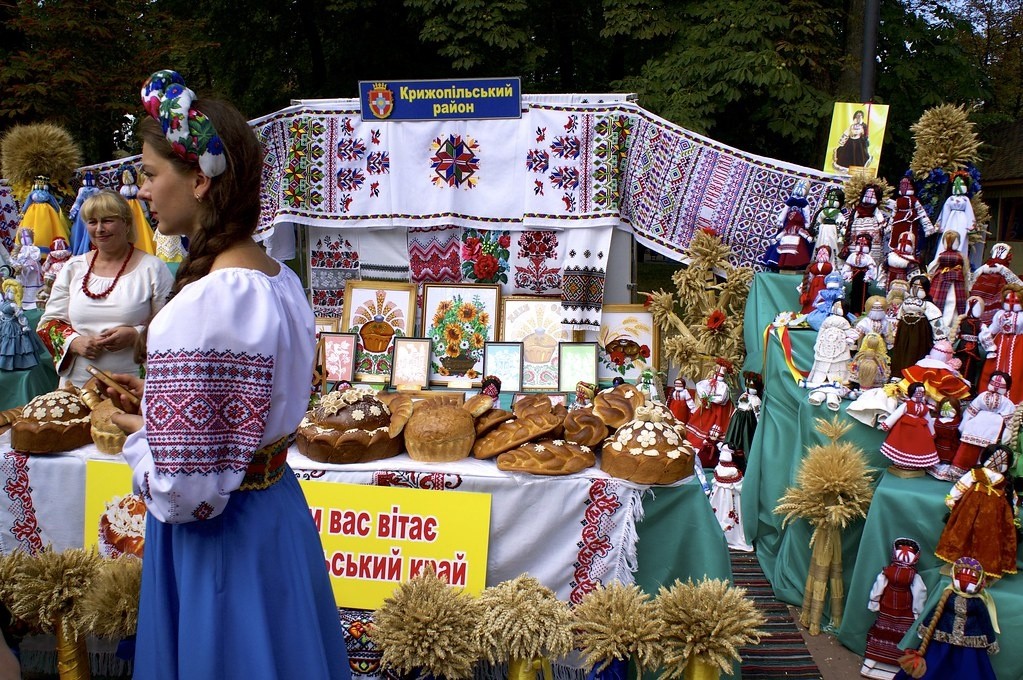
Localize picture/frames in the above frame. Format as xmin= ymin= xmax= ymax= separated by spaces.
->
xmin=420 ymin=282 xmax=501 ymax=387
xmin=483 ymin=341 xmax=524 ymax=393
xmin=340 ymin=280 xmax=418 ymax=382
xmin=409 ymin=392 xmax=465 ymax=402
xmin=389 ymin=337 xmax=432 ymax=390
xmin=558 ymin=342 xmax=598 ymax=392
xmin=498 ymin=296 xmax=578 ymax=393
xmin=578 ymin=304 xmax=660 ymax=382
xmin=315 ymin=318 xmax=340 ymax=343
xmin=315 ymin=332 xmax=358 ymax=383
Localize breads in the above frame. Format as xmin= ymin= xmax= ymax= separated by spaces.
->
xmin=296 ymin=390 xmax=696 ymax=486
xmin=0 ymin=368 xmax=133 ymax=456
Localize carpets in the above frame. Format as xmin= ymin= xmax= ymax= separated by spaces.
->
xmin=728 ymin=547 xmax=824 ymax=680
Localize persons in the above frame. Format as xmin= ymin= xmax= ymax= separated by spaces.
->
xmin=36 ymin=189 xmax=175 ymax=397
xmin=671 ymin=168 xmax=1023 ymax=680
xmin=0 ymin=164 xmax=151 ymax=372
xmin=106 ymin=70 xmax=353 ymax=680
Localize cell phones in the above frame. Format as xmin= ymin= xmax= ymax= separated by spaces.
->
xmin=85 ymin=363 xmax=141 ymax=406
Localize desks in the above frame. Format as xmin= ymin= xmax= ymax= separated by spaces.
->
xmin=0 ymin=428 xmax=742 ymax=680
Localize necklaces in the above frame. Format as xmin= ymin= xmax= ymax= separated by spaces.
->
xmin=82 ymin=241 xmax=135 ymax=299
